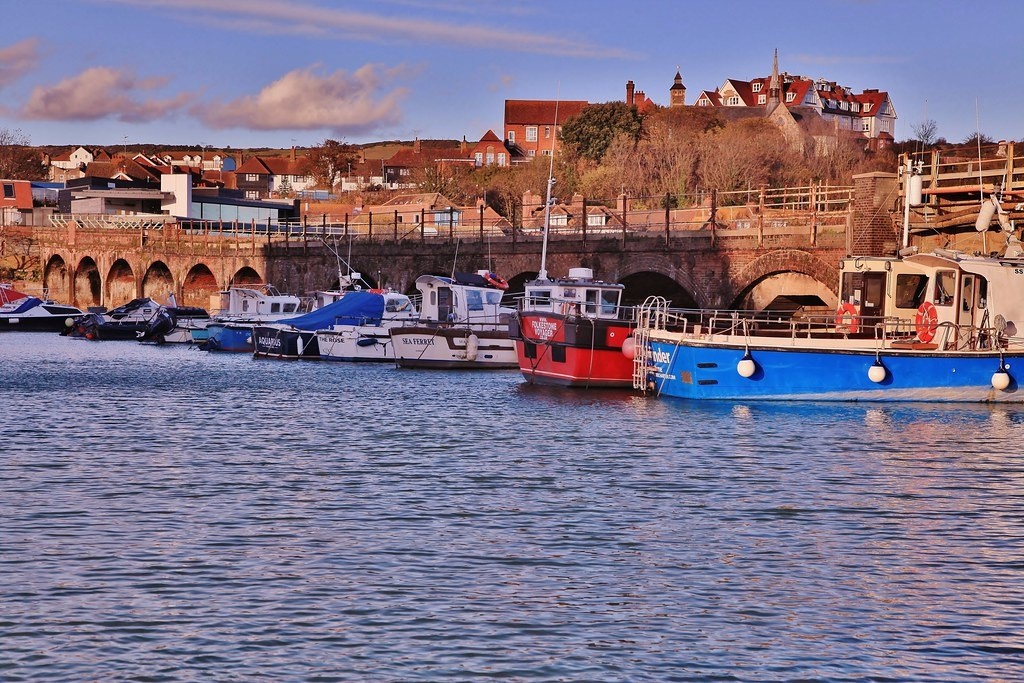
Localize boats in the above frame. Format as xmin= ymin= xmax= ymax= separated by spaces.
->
xmin=507 ymin=158 xmax=930 ymax=388
xmin=389 ymin=100 xmax=624 ymax=371
xmin=0 ymin=217 xmax=519 ymax=363
xmin=622 ymin=94 xmax=1024 ymax=403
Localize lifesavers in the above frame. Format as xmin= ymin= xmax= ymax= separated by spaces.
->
xmin=915 ymin=302 xmax=938 ymax=343
xmin=366 ymin=289 xmax=389 ymax=294
xmin=485 ymin=272 xmax=509 ymax=289
xmin=836 ymin=303 xmax=858 ymax=333
